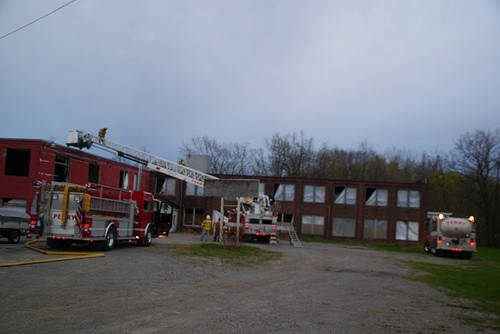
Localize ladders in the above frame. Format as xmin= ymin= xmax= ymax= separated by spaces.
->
xmin=84 ymin=194 xmax=133 ymax=216
xmin=286 ymin=223 xmax=303 ymax=248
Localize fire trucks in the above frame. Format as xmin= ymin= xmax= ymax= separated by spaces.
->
xmin=66 ymin=129 xmax=278 ymax=245
xmin=424 ymin=212 xmax=477 ymax=258
xmin=26 ymin=178 xmax=175 ymax=252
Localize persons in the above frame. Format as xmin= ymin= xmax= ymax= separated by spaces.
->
xmin=200 ymin=214 xmax=212 ymax=241
xmin=178 ymin=159 xmax=187 ymax=173
xmin=98 ymin=126 xmax=108 ymax=144
xmin=213 ymin=218 xmax=220 ymax=241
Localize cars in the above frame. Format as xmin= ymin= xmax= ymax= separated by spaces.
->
xmin=0 ymin=198 xmax=30 ymax=243
